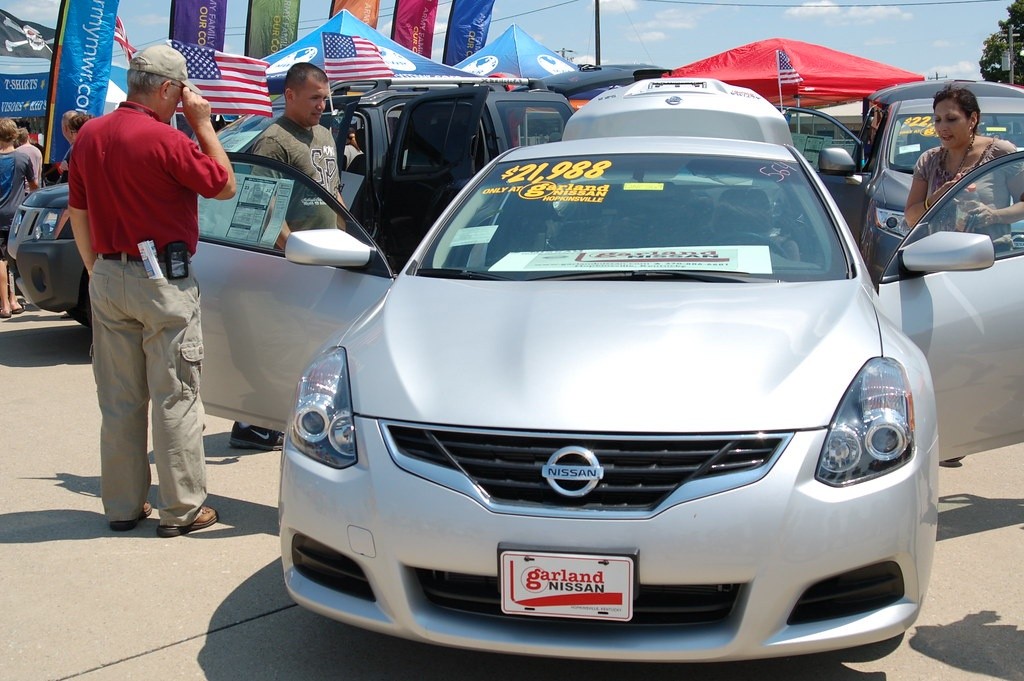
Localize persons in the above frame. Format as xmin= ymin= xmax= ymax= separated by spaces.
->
xmin=248 ymin=62 xmax=346 ymax=250
xmin=70 ymin=45 xmax=237 ymax=537
xmin=0 ymin=110 xmax=96 ymax=320
xmin=906 ymin=89 xmax=1024 ymax=254
xmin=345 ymin=126 xmax=364 ymax=154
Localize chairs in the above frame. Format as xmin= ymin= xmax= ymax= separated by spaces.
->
xmin=485 ymin=184 xmax=770 ymax=267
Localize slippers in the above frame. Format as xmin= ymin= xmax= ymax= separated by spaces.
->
xmin=11 ymin=302 xmax=25 ymax=313
xmin=0 ymin=308 xmax=12 ymax=318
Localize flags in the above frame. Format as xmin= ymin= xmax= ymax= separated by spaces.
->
xmin=776 ymin=51 xmax=804 ymax=86
xmin=0 ymin=9 xmax=56 ymax=61
xmin=43 ymin=0 xmax=119 ymax=164
xmin=245 ymin=0 xmax=300 ymax=59
xmin=329 ymin=0 xmax=380 ymax=30
xmin=442 ymin=0 xmax=495 ymax=67
xmin=114 ymin=16 xmax=137 ymax=67
xmin=166 ymin=39 xmax=273 ymax=117
xmin=391 ymin=0 xmax=438 ymax=59
xmin=321 ymin=32 xmax=395 ymax=80
xmin=169 ymin=0 xmax=227 ymax=52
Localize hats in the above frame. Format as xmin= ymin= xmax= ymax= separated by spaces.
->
xmin=131 ymin=44 xmax=203 ymax=95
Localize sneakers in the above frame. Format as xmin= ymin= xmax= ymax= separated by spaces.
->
xmin=229 ymin=421 xmax=285 ymax=450
xmin=110 ymin=501 xmax=152 ymax=531
xmin=157 ymin=506 xmax=219 ymax=537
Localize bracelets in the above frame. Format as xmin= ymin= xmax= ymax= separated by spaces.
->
xmin=924 ymin=199 xmax=929 ymax=210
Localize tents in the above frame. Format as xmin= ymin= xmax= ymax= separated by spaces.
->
xmin=259 ymin=9 xmax=610 ymax=98
xmin=661 ymin=37 xmax=925 ymax=134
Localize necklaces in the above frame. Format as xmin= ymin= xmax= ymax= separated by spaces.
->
xmin=941 ymin=139 xmax=974 ymax=181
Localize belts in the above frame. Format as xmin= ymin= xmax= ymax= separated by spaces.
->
xmin=97 ymin=253 xmax=143 ymax=262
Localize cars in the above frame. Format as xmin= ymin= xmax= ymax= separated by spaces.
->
xmin=190 ymin=135 xmax=1024 ymax=663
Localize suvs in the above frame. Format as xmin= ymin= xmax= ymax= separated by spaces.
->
xmin=6 ymin=64 xmax=676 ymax=329
xmin=562 ymin=79 xmax=1024 ymax=295
